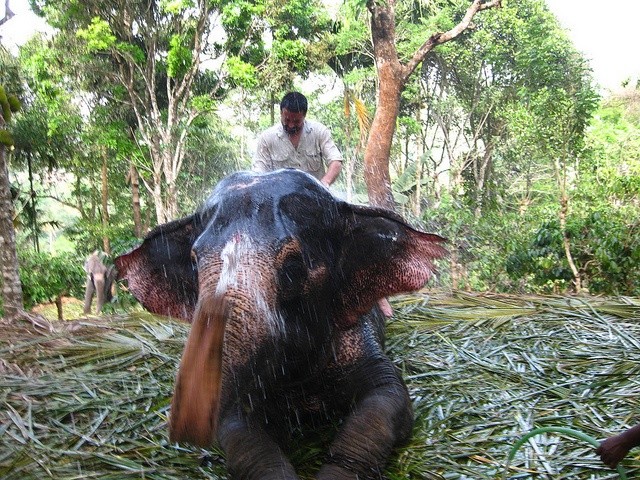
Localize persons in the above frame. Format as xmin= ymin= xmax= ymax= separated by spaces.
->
xmin=252 ymin=91 xmax=343 ymax=191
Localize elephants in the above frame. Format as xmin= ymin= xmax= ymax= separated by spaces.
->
xmin=81 ymin=164 xmax=452 ymax=480
xmin=79 ymin=249 xmax=120 ymax=316
